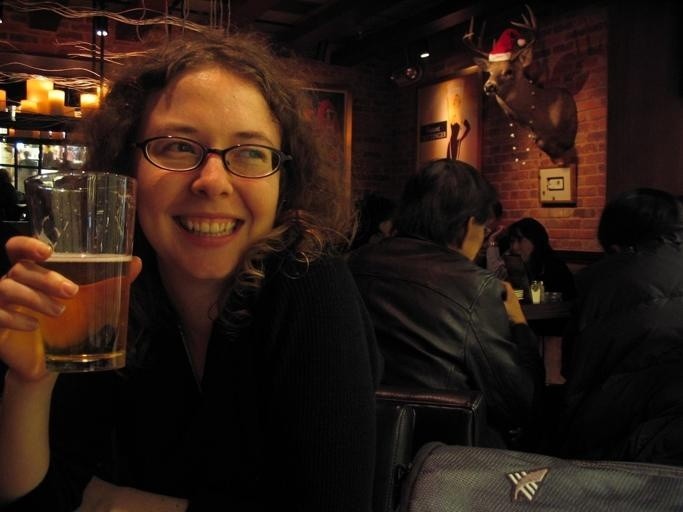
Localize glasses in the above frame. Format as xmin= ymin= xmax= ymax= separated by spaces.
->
xmin=130 ymin=135 xmax=293 ymax=179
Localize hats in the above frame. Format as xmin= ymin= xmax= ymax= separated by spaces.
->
xmin=489 ymin=28 xmax=526 ymax=63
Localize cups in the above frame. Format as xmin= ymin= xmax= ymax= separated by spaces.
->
xmin=22 ymin=171 xmax=138 ymax=375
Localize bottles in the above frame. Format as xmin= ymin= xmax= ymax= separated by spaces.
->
xmin=530 ymin=280 xmax=545 ymax=306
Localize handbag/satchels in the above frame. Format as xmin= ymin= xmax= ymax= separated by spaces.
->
xmin=396 ymin=440 xmax=682 ymax=511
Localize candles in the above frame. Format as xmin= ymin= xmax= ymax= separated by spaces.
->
xmin=1 ymin=74 xmax=100 ymax=116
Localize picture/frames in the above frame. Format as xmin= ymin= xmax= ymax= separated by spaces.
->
xmin=300 ymin=87 xmax=352 ymax=216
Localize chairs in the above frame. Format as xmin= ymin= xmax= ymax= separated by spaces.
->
xmin=374 ymin=384 xmax=683 ymax=510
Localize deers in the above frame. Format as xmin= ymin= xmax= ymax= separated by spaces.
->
xmin=462 ymin=3 xmax=577 ymax=161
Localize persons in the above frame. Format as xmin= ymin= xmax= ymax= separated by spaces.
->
xmin=339 ymin=159 xmax=682 ymax=473
xmin=0 ymin=149 xmax=91 ymax=243
xmin=0 ymin=22 xmax=383 ymax=511
